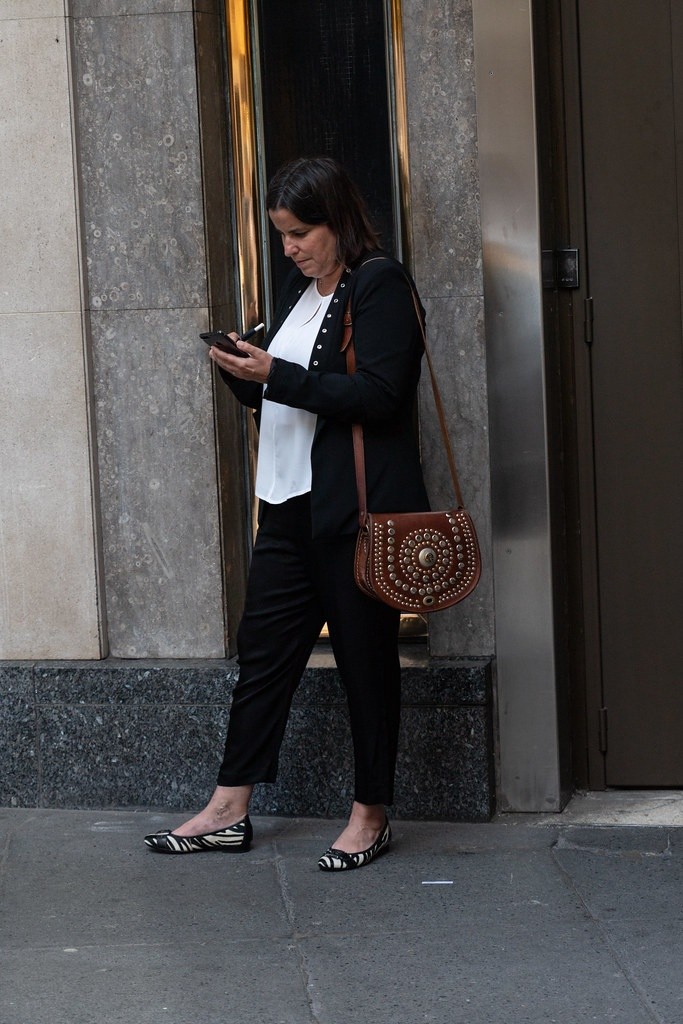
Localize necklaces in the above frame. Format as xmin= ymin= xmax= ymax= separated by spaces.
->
xmin=319 ymin=279 xmax=334 ymax=295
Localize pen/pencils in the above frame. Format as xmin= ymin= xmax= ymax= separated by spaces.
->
xmin=236 ymin=323 xmax=265 ymax=342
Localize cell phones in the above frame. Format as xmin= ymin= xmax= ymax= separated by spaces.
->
xmin=199 ymin=330 xmax=250 ymax=358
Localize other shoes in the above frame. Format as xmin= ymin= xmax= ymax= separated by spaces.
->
xmin=319 ymin=814 xmax=392 ymax=869
xmin=145 ymin=814 xmax=253 ymax=852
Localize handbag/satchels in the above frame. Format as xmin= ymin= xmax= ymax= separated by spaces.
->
xmin=353 ymin=510 xmax=482 ymax=613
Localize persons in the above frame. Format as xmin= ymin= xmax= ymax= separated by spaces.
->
xmin=143 ymin=156 xmax=427 ymax=870
